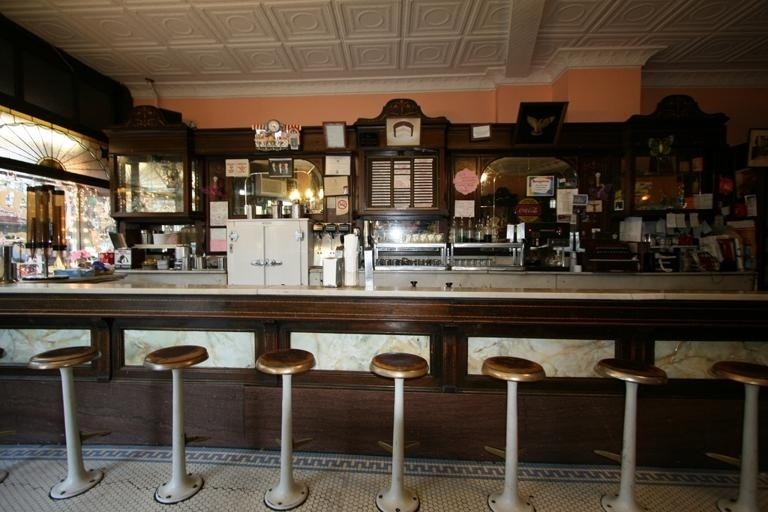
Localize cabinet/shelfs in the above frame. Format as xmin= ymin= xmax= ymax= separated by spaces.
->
xmin=374 ymin=243 xmax=525 ymax=271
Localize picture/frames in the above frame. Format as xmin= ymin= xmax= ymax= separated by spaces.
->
xmin=513 ymin=101 xmax=569 ymax=147
xmin=322 ymin=121 xmax=348 ymax=152
xmin=385 ymin=114 xmax=423 ymax=149
xmin=745 ymin=127 xmax=768 ymax=168
xmin=470 ymin=123 xmax=493 ymax=142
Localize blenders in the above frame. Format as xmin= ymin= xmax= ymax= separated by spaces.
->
xmin=309 ymin=222 xmax=355 ymax=287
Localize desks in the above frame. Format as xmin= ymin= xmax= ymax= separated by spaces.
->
xmin=0 ymin=282 xmax=768 ymax=393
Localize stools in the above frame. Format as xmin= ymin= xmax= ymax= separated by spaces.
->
xmin=481 ymin=355 xmax=546 ymax=512
xmin=712 ymin=360 xmax=768 ymax=510
xmin=593 ymin=358 xmax=668 ymax=508
xmin=255 ymin=348 xmax=316 ymax=509
xmin=27 ymin=345 xmax=104 ymax=500
xmin=369 ymin=352 xmax=429 ymax=512
xmin=143 ymin=345 xmax=209 ymax=503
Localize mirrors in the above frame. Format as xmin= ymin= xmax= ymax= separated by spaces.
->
xmin=232 ymin=158 xmax=324 ymax=216
xmin=633 ymin=175 xmax=684 ymax=209
xmin=115 ymin=154 xmax=204 ymax=212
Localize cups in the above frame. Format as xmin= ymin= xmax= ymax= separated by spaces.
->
xmin=402 ymin=258 xmax=408 ymax=265
xmin=434 ymin=259 xmax=441 ymax=265
xmin=418 ymin=259 xmax=425 ymax=266
xmin=393 ymin=259 xmax=399 ymax=265
xmin=475 ymin=259 xmax=481 ymax=266
xmin=462 ymin=258 xmax=467 ymax=266
xmin=487 ymin=259 xmax=493 ymax=267
xmin=455 ymin=259 xmax=461 ymax=265
xmin=467 ymin=259 xmax=474 ymax=267
xmin=481 ymin=259 xmax=487 ymax=267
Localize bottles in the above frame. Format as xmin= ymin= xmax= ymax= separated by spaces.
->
xmin=450 ymin=219 xmax=458 ymax=243
xmin=466 ymin=219 xmax=474 ymax=243
xmin=484 ymin=215 xmax=492 ymax=242
xmin=474 ymin=217 xmax=484 ymax=243
xmin=458 ymin=220 xmax=466 ymax=242
